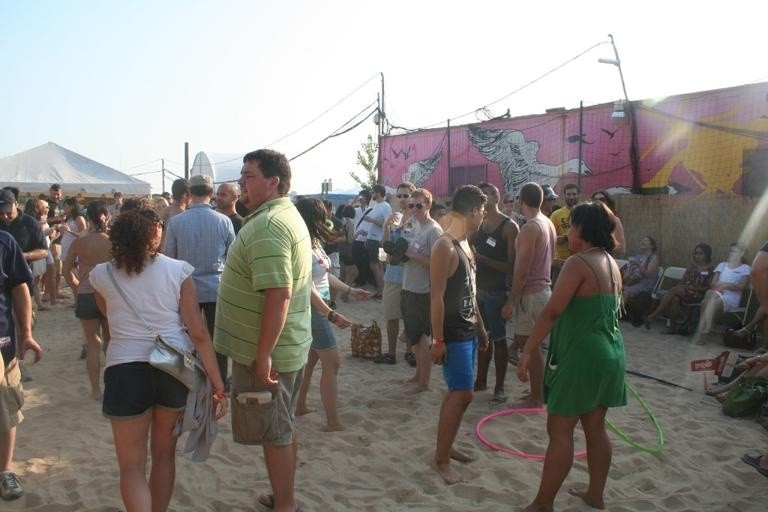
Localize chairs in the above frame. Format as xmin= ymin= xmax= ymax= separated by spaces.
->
xmin=726 ymin=282 xmax=753 ymax=326
xmin=652 ymin=266 xmax=686 ymax=325
xmin=652 ymin=266 xmax=663 ymax=293
xmin=680 ymin=301 xmax=701 ymax=337
xmin=613 ymin=259 xmax=629 ymax=276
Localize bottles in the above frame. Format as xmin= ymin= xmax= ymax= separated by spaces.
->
xmin=22 ymin=348 xmax=36 ymax=370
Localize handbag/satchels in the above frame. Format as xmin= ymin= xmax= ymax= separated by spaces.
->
xmin=148 ymin=331 xmax=209 ymax=395
xmin=619 ymin=260 xmax=643 ymax=286
xmin=350 ymin=319 xmax=383 ymax=360
xmin=722 ymin=327 xmax=757 ymax=351
xmin=722 ymin=375 xmax=767 ymax=419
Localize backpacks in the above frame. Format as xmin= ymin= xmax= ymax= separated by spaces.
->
xmin=627 ymin=288 xmax=653 ymax=328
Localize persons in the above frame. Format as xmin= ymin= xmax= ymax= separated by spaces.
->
xmin=88 ymin=209 xmax=229 ymax=512
xmin=429 ymin=185 xmax=489 ymax=484
xmin=151 ymin=173 xmax=245 ymax=390
xmin=470 ymin=181 xmax=627 ymax=408
xmin=294 ymin=182 xmax=446 ymax=432
xmin=705 ymin=351 xmax=767 ymax=402
xmin=743 ymin=240 xmax=768 ymax=481
xmin=1 ymin=181 xmax=149 ymax=398
xmin=621 ymin=235 xmax=751 ymax=347
xmin=0 ymin=226 xmax=43 ymax=501
xmin=213 ymin=149 xmax=313 ymax=512
xmin=515 ymin=203 xmax=627 ymax=512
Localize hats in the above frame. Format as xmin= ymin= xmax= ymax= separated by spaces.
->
xmin=0 ymin=189 xmax=16 ymax=212
xmin=188 ymin=174 xmax=214 ymax=189
xmin=536 ymin=183 xmax=560 ymax=200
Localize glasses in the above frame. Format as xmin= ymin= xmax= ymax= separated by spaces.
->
xmin=729 ymin=249 xmax=738 ymax=253
xmin=516 ymin=195 xmax=521 ymax=200
xmin=503 ymin=200 xmax=514 ymax=204
xmin=693 ymin=252 xmax=704 ymax=255
xmin=591 ymin=197 xmax=609 ymax=202
xmin=408 ymin=202 xmax=428 ymax=210
xmin=395 ymin=194 xmax=409 ymax=199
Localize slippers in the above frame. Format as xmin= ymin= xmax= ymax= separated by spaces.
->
xmin=695 ymin=339 xmax=706 ymax=346
xmin=740 ymin=452 xmax=768 ymax=479
xmin=701 ymin=328 xmax=714 ymax=336
xmin=255 ymin=494 xmax=304 ymax=512
xmin=641 ymin=313 xmax=653 ymax=331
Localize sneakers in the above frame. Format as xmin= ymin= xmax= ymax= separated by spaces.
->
xmin=1 ymin=471 xmax=25 ymax=501
xmin=404 ymin=351 xmax=417 ymax=368
xmin=372 ymin=352 xmax=397 ymax=364
xmin=382 ymin=237 xmax=410 ymax=266
xmin=17 ymin=360 xmax=34 ymax=383
xmin=733 ymin=326 xmax=752 ymax=338
xmin=79 ymin=344 xmax=89 ymax=360
xmin=368 ymin=293 xmax=383 ymax=303
xmin=328 ymin=301 xmax=337 ymax=310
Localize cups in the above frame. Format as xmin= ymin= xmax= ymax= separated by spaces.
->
xmin=392 ymin=211 xmax=404 ymax=226
